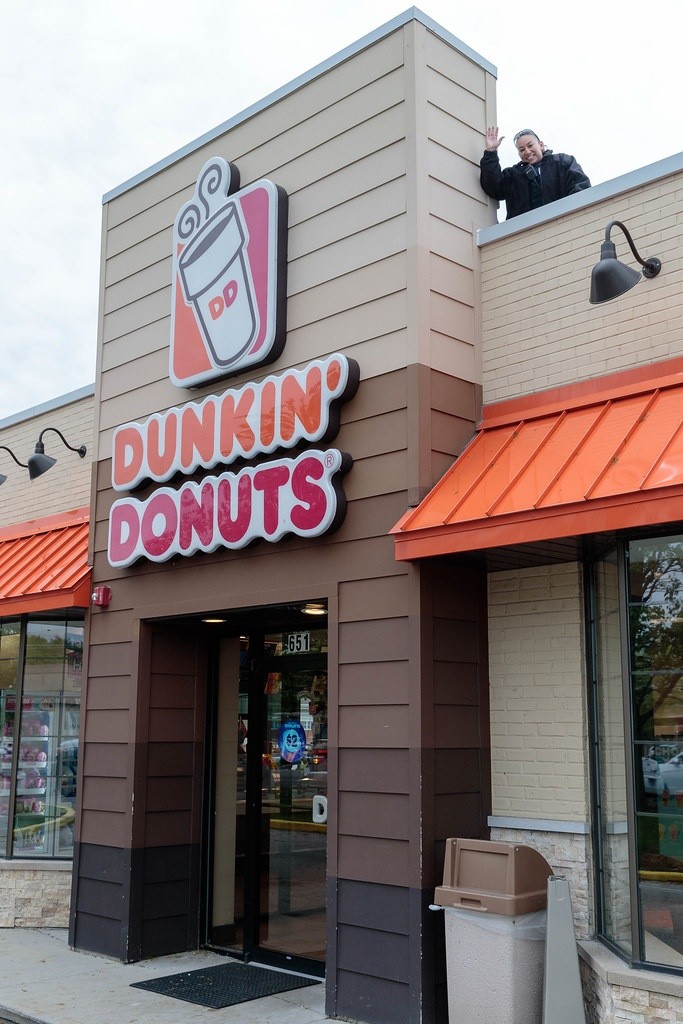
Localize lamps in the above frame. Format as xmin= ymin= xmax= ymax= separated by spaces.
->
xmin=589 ymin=219 xmax=662 ymax=305
xmin=28 ymin=428 xmax=87 ymax=480
xmin=0 ymin=445 xmax=29 ymax=486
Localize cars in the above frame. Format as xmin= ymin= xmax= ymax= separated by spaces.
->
xmin=641 ymin=742 xmax=682 ymax=797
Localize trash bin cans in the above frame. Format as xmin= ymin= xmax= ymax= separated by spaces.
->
xmin=434 ymin=836 xmax=556 ymax=1024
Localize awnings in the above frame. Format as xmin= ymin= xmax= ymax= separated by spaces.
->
xmin=0 ymin=517 xmax=91 ymax=620
xmin=389 ymin=374 xmax=683 ymax=573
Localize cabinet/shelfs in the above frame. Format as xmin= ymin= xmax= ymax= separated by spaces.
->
xmin=0 ymin=689 xmax=81 ymax=858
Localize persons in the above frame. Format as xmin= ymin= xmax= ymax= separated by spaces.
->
xmin=480 ymin=126 xmax=591 ymax=221
